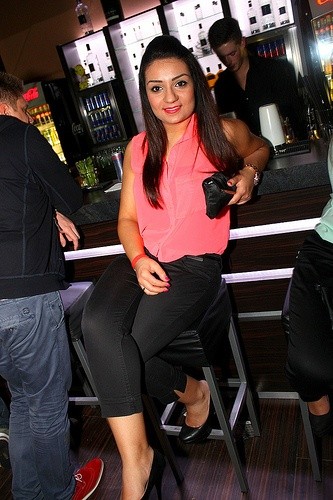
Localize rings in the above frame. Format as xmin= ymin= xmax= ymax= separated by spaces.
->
xmin=142 ymin=288 xmax=145 ymax=292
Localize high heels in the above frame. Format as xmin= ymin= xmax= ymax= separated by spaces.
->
xmin=178 ymin=379 xmax=218 ymax=444
xmin=118 ymin=446 xmax=166 ymax=500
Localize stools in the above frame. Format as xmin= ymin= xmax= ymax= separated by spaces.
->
xmin=49 ymin=280 xmax=101 ymax=451
xmin=146 ymin=277 xmax=261 ymax=500
xmin=281 ymin=263 xmax=333 ymax=480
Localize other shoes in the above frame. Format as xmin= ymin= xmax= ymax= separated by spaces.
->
xmin=308 ymin=390 xmax=333 ymax=437
xmin=71 ymin=457 xmax=105 ymax=500
xmin=0 ymin=427 xmax=11 ymax=470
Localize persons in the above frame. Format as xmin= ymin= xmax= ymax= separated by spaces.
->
xmin=208 ymin=18 xmax=312 ymax=141
xmin=0 ymin=75 xmax=105 ymax=500
xmin=81 ymin=34 xmax=273 ymax=500
xmin=282 ymin=139 xmax=333 ymax=462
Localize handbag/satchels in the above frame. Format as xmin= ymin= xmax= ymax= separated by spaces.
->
xmin=202 ymin=156 xmax=244 ymax=219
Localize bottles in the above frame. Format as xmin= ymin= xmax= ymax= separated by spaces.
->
xmin=215 ymin=64 xmax=224 ymax=79
xmin=26 ymin=104 xmax=68 ymax=165
xmin=104 ymin=51 xmax=116 ymax=80
xmin=197 ymin=24 xmax=211 ymax=56
xmin=205 ymin=67 xmax=216 ymax=91
xmin=186 ymin=34 xmax=195 ymax=58
xmin=277 ymin=0 xmax=290 ymax=26
xmin=257 ymin=38 xmax=286 ymax=59
xmin=75 ymin=0 xmax=94 ymax=36
xmin=259 ymin=0 xmax=277 ymax=30
xmin=247 ymin=0 xmax=260 ymax=35
xmin=100 ymin=0 xmax=124 ymax=26
xmin=85 ymin=43 xmax=104 ymax=84
xmin=132 ymin=43 xmax=146 ymax=78
xmin=83 ymin=63 xmax=93 ymax=87
xmin=313 ymin=15 xmax=333 ymax=108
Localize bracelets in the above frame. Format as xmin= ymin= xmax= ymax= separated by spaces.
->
xmin=131 ymin=253 xmax=146 ymax=271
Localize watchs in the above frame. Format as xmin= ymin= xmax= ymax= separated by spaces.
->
xmin=244 ymin=163 xmax=261 ymax=186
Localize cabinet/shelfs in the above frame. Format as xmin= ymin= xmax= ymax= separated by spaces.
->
xmin=55 ymin=0 xmax=323 ymax=171
